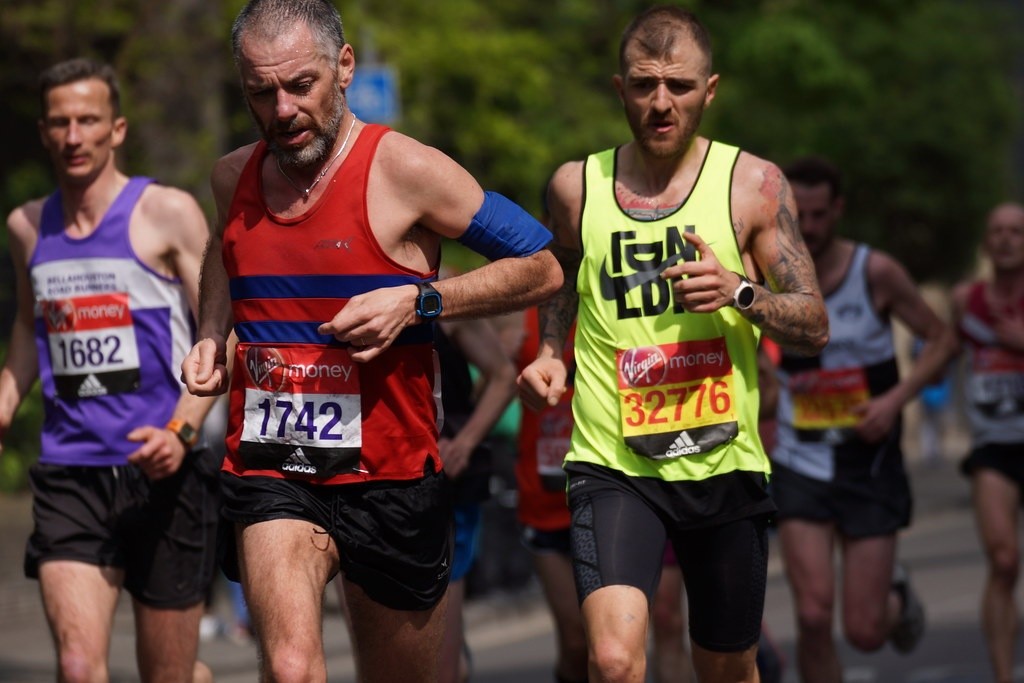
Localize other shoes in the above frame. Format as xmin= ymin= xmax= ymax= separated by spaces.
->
xmin=890 ymin=563 xmax=924 ymax=656
xmin=756 ymin=620 xmax=785 ymax=683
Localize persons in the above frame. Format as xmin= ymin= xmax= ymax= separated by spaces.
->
xmin=431 ymin=317 xmax=519 ymax=682
xmin=755 ymin=160 xmax=957 ymax=682
xmin=907 ymin=283 xmax=955 ymax=458
xmin=517 ymin=9 xmax=829 ymax=682
xmin=951 ymin=196 xmax=1024 ymax=683
xmin=180 ymin=0 xmax=565 ymax=683
xmin=506 ymin=304 xmax=688 ymax=682
xmin=0 ymin=66 xmax=237 ymax=683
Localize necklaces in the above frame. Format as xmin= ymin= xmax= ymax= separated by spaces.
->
xmin=276 ymin=114 xmax=356 ymax=199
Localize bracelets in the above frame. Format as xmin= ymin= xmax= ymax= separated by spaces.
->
xmin=167 ymin=418 xmax=197 ymax=447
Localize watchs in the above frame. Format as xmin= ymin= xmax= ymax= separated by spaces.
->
xmin=413 ymin=281 xmax=443 ymax=329
xmin=732 ymin=273 xmax=756 ymax=311
